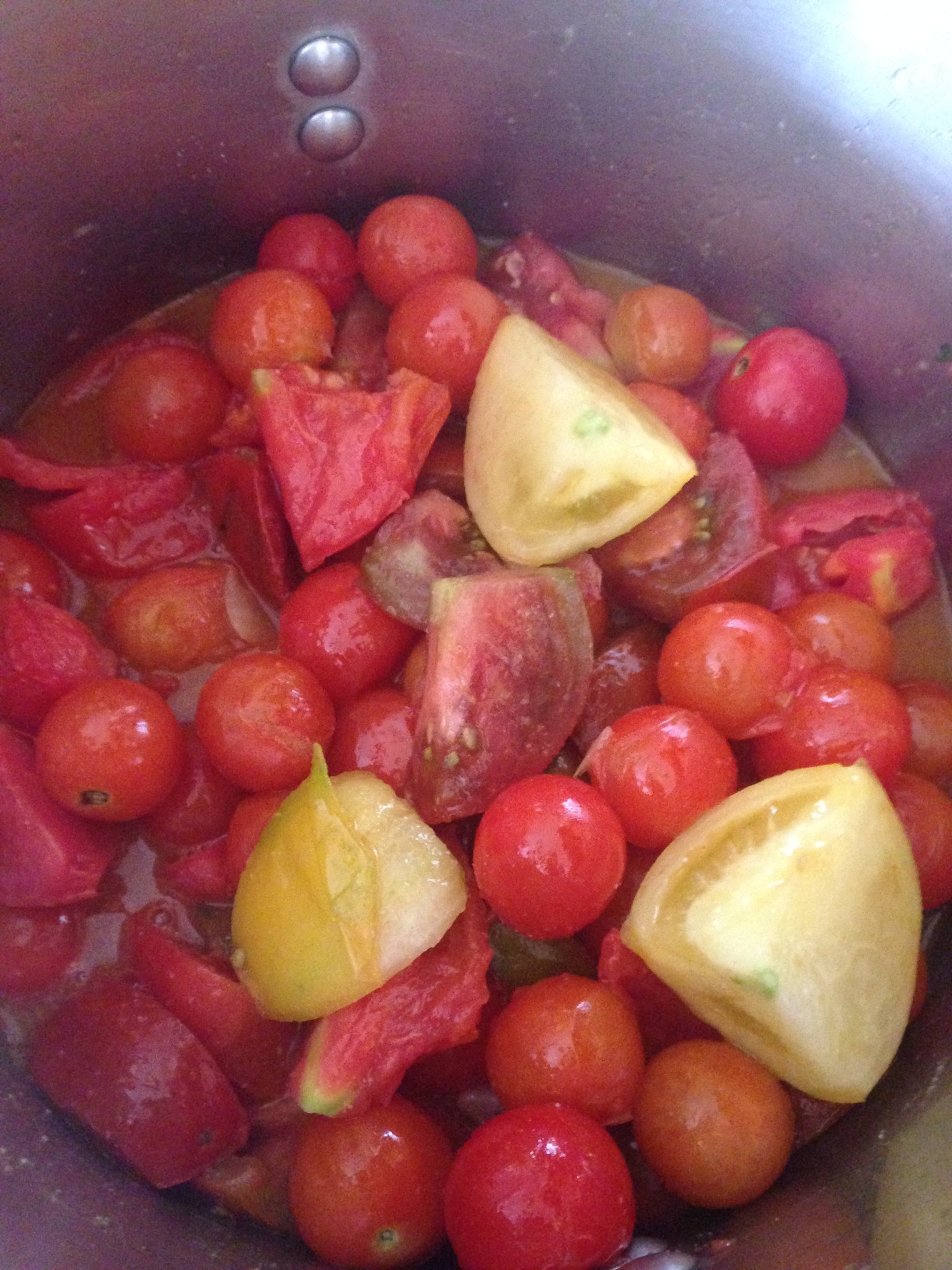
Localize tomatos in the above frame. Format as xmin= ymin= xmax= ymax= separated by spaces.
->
xmin=0 ymin=187 xmax=948 ymax=1270
